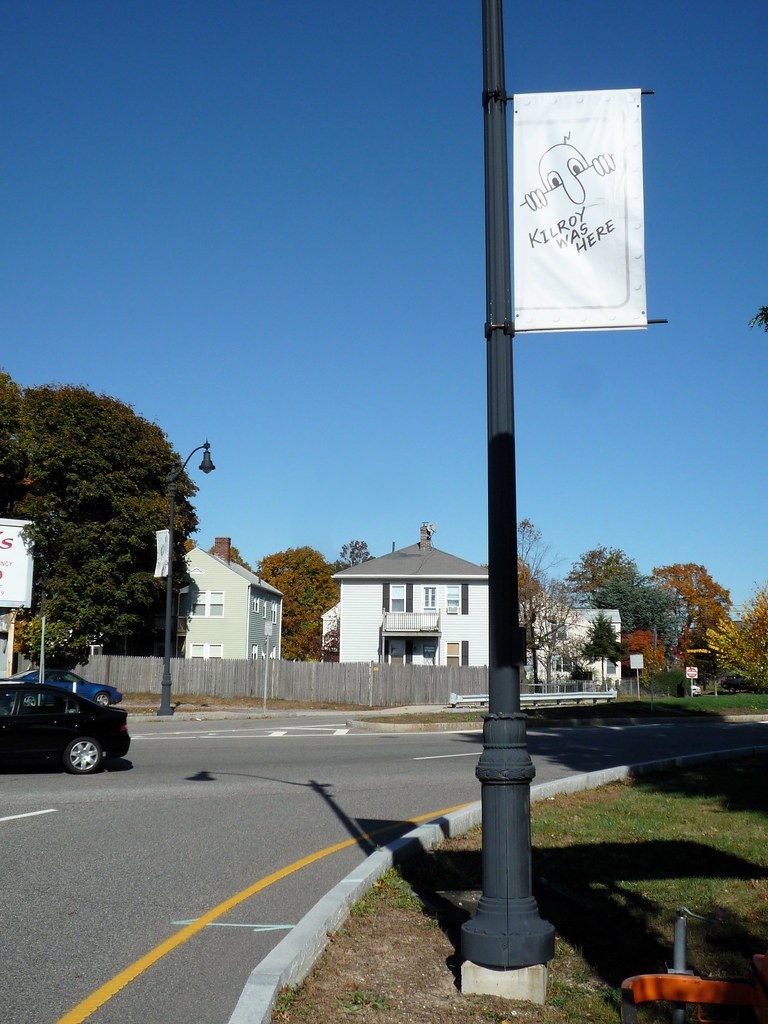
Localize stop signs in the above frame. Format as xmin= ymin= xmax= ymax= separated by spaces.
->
xmin=687 ymin=668 xmax=698 ymax=678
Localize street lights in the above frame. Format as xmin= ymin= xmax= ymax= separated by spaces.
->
xmin=157 ymin=437 xmax=216 ymax=718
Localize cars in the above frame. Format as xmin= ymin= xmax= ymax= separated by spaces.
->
xmin=4 ymin=668 xmax=125 ymax=707
xmin=692 ymin=686 xmax=702 ymax=697
xmin=0 ymin=679 xmax=131 ymax=775
xmin=721 ymin=674 xmax=758 ymax=694
